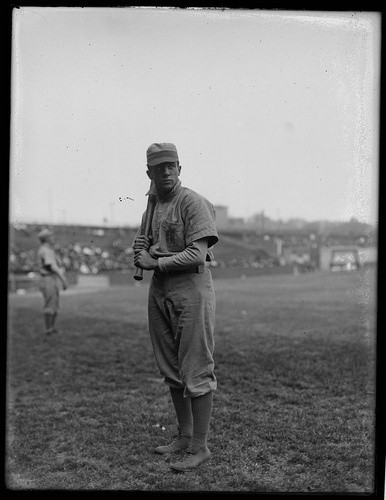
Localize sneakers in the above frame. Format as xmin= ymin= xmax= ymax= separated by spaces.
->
xmin=169 ymin=445 xmax=211 ymax=471
xmin=154 ymin=433 xmax=192 ymax=455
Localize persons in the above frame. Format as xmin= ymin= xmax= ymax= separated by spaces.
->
xmin=133 ymin=143 xmax=218 ymax=471
xmin=36 ymin=228 xmax=68 ymax=334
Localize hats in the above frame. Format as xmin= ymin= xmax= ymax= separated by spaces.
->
xmin=38 ymin=229 xmax=52 ymax=239
xmin=146 ymin=143 xmax=178 ymax=167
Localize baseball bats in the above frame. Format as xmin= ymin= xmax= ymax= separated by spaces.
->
xmin=134 ymin=180 xmax=156 ymax=280
